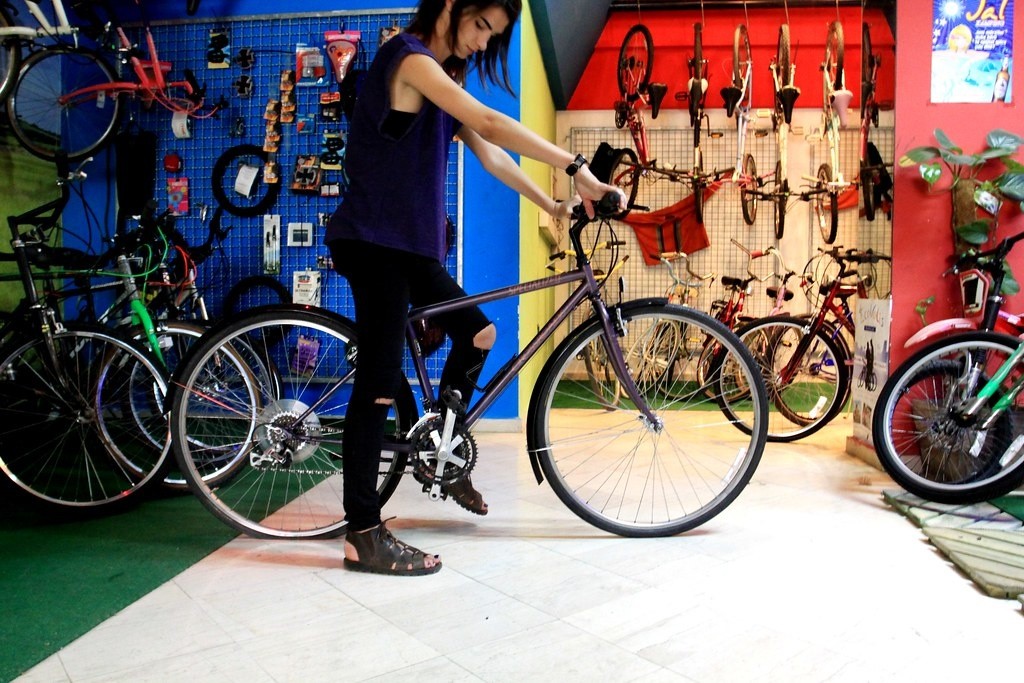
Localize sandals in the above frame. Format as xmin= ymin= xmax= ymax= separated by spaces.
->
xmin=412 ymin=465 xmax=489 ymax=516
xmin=343 ymin=516 xmax=443 ymax=577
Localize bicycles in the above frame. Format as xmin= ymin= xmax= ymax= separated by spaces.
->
xmin=544 ymin=21 xmax=894 ymax=445
xmin=888 ymin=225 xmax=1024 ymax=486
xmin=163 ymin=193 xmax=773 ymax=539
xmin=872 ymin=333 xmax=1024 ymax=507
xmin=0 ymin=0 xmax=284 ymax=516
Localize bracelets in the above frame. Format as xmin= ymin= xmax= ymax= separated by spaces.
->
xmin=565 ymin=153 xmax=588 ymax=176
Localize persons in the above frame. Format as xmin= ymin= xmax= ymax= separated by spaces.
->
xmin=327 ymin=1 xmax=625 ymax=576
xmin=853 ymin=340 xmax=874 ymax=428
